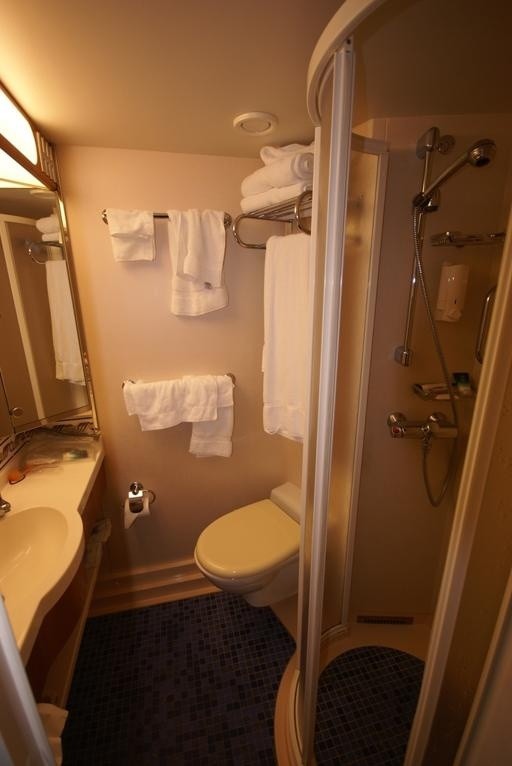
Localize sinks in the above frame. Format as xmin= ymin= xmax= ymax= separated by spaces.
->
xmin=0 ymin=502 xmax=83 ymax=647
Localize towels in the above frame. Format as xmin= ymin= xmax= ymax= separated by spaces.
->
xmin=107 ymin=207 xmax=157 ymax=265
xmin=187 ymin=376 xmax=237 ymax=458
xmin=238 ymin=182 xmax=313 ymax=225
xmin=163 ymin=211 xmax=229 ymax=317
xmin=241 ymin=143 xmax=311 ymax=198
xmin=260 ymin=232 xmax=313 ymax=446
xmin=34 ymin=213 xmax=86 ymax=382
xmin=121 ymin=374 xmax=217 ymax=432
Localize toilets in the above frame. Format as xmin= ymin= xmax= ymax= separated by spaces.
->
xmin=195 ymin=480 xmax=301 ymax=610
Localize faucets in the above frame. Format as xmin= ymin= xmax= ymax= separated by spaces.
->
xmin=1 ymin=496 xmax=10 ymax=510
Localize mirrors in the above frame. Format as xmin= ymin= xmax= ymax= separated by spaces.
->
xmin=0 ymin=187 xmax=95 ymax=443
